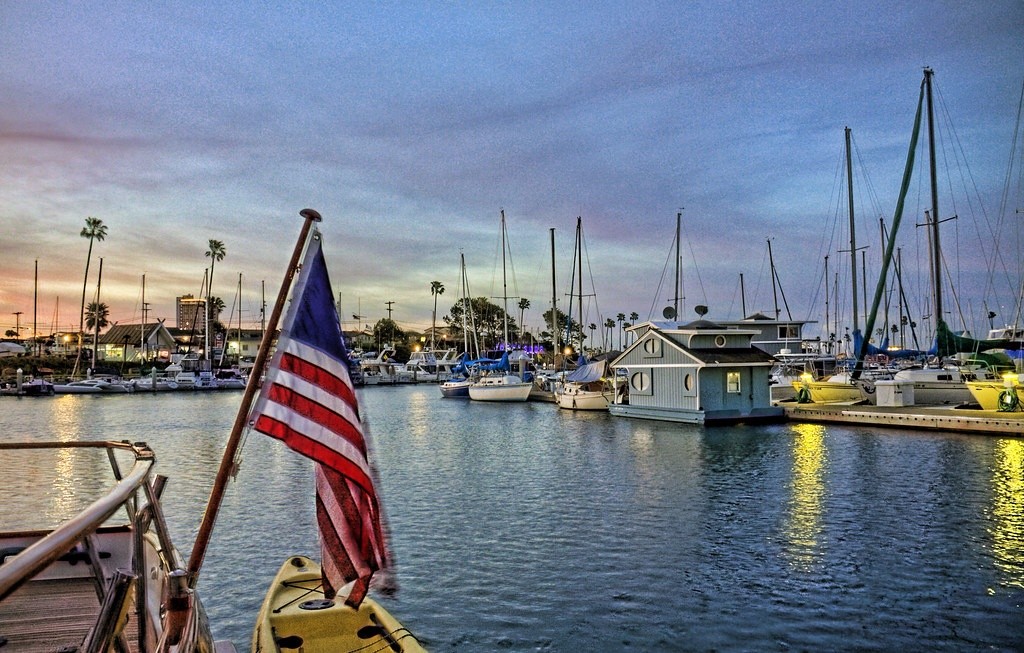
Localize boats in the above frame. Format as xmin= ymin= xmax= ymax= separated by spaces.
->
xmin=726 ymin=66 xmax=1024 ymax=411
xmin=0 ymin=207 xmax=388 ymax=653
xmin=250 ymin=551 xmax=429 ymax=652
xmin=349 ymin=208 xmax=715 ymax=411
xmin=1 ymin=256 xmax=266 ymax=397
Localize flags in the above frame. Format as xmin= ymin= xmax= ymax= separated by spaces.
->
xmin=244 ymin=229 xmax=387 ymax=611
